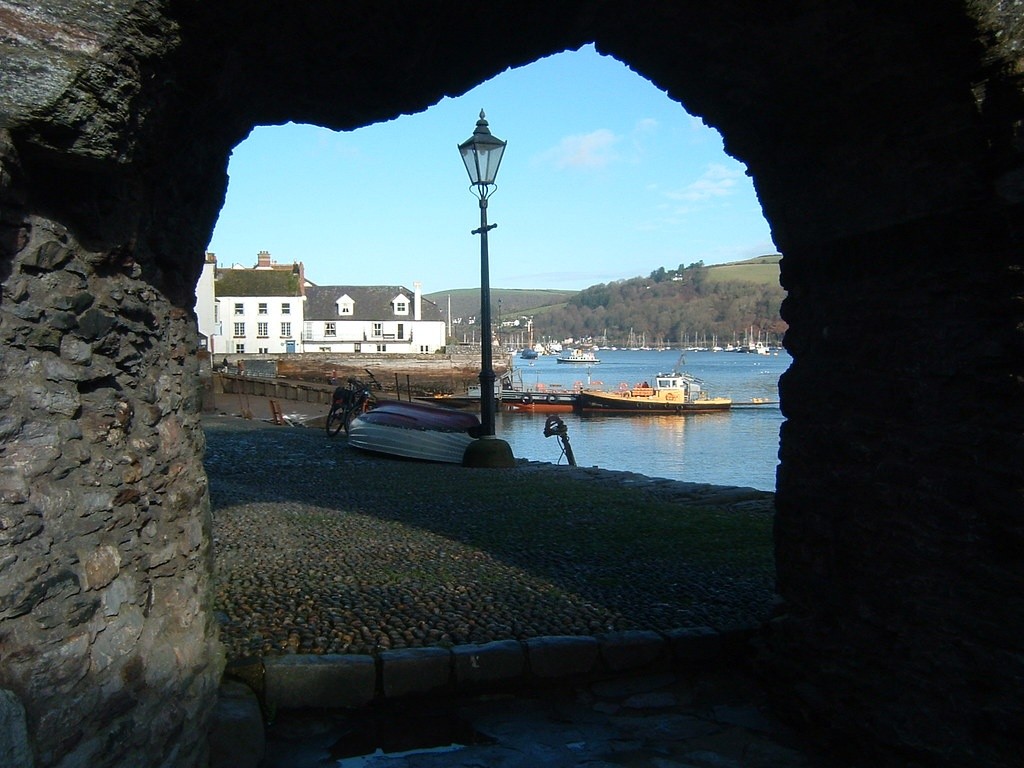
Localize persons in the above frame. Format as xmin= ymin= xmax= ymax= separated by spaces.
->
xmin=637 ymin=382 xmax=649 ymax=388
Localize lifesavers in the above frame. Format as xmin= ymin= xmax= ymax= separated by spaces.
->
xmin=665 ymin=393 xmax=674 ymax=401
xmin=573 ymin=382 xmax=582 ymax=389
xmin=664 ymin=402 xmax=671 ymax=409
xmin=636 ymin=401 xmax=644 ymax=409
xmin=619 ymin=383 xmax=627 ymax=390
xmin=675 ymin=404 xmax=684 ymax=411
xmin=648 ymin=402 xmax=656 ymax=409
xmin=623 ymin=392 xmax=630 ymax=399
xmin=521 ymin=393 xmax=534 ymax=404
xmin=547 ymin=393 xmax=559 ymax=404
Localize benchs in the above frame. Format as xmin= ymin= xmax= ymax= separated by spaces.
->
xmin=632 ymin=387 xmax=656 ymax=397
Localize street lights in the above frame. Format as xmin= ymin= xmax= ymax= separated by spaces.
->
xmin=456 ymin=107 xmax=508 ymax=469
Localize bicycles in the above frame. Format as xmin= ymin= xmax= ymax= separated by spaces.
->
xmin=326 ymin=395 xmax=391 ymax=439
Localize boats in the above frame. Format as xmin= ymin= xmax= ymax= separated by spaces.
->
xmin=347 ymin=400 xmax=482 ymax=464
xmin=713 ymin=325 xmax=769 ymax=355
xmin=557 ymin=348 xmax=602 ymax=365
xmin=531 ymin=339 xmax=564 ymax=354
xmin=576 ymin=374 xmax=735 ymax=414
xmin=596 ymin=326 xmax=707 ymax=351
